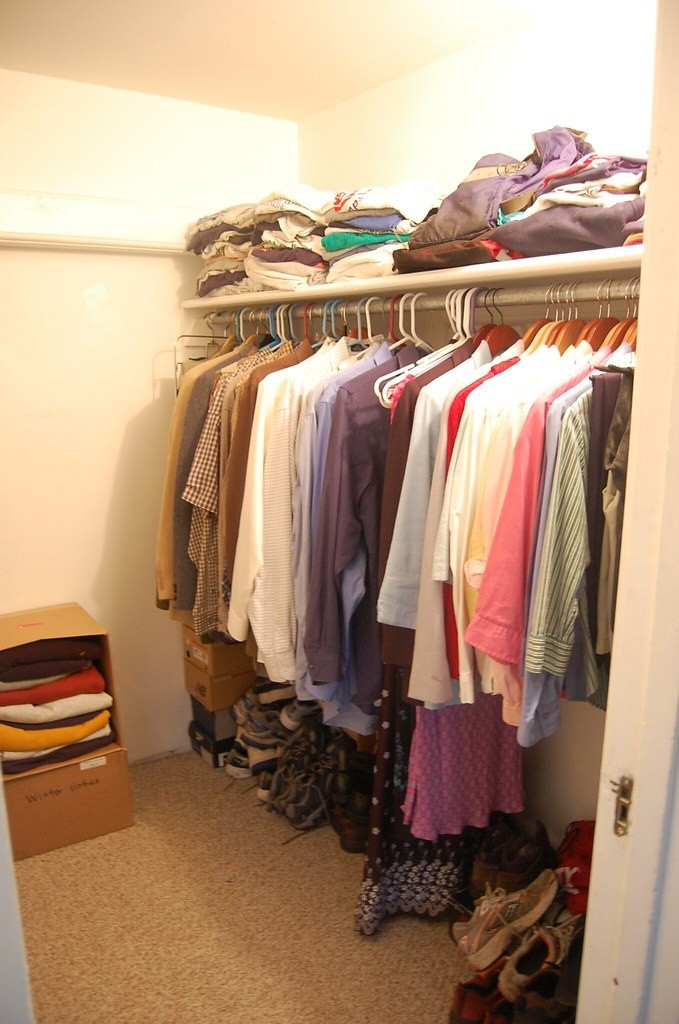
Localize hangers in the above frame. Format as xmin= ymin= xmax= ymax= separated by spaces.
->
xmin=347 ymin=297 xmax=368 ymax=349
xmin=310 ymin=300 xmax=337 ymax=349
xmin=343 ymin=299 xmax=374 ymax=340
xmin=329 ymin=299 xmax=342 ymax=342
xmin=271 ymin=304 xmax=287 ymax=352
xmin=286 ymin=304 xmax=294 ymax=341
xmin=276 ymin=305 xmax=282 ymax=339
xmin=258 ymin=308 xmax=275 ymax=349
xmin=303 ymin=301 xmax=317 ymax=344
xmin=259 ymin=305 xmax=291 ymax=351
xmin=354 ymin=296 xmax=382 ymax=357
xmin=287 ymin=302 xmax=302 ymax=349
xmin=374 ymin=276 xmax=640 ymax=409
xmin=175 ymin=306 xmax=262 ymax=364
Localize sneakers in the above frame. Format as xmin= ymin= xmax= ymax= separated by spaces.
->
xmin=452 ymin=869 xmax=559 ymax=971
xmin=498 ymin=913 xmax=581 ymax=1001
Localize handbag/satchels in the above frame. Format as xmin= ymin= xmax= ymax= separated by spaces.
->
xmin=558 ymin=819 xmax=595 ymax=913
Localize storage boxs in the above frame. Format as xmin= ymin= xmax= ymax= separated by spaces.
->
xmin=182 ymin=624 xmax=249 ymax=676
xmin=190 ymin=693 xmax=236 ymax=742
xmin=183 ymin=658 xmax=255 ymax=713
xmin=0 ymin=600 xmax=136 ymax=862
xmin=188 ymin=720 xmax=235 ymax=769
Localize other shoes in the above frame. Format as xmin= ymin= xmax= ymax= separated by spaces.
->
xmin=514 ymin=926 xmax=585 ymax=1024
xmin=449 ymin=956 xmax=515 ymax=1024
xmin=224 ymin=677 xmax=372 ymax=853
xmin=469 ymin=822 xmax=556 ymax=899
